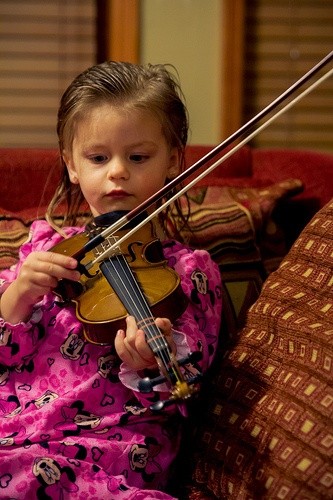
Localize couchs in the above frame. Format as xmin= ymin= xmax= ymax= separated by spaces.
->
xmin=0 ymin=147 xmax=333 ymax=500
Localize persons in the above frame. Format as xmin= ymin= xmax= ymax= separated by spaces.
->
xmin=0 ymin=60 xmax=223 ymax=499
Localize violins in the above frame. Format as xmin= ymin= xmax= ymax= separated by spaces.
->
xmin=46 ymin=209 xmax=206 ymax=415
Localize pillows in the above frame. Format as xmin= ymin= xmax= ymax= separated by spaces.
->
xmin=0 ymin=175 xmax=304 ymax=354
xmin=169 ymin=198 xmax=333 ymax=500
